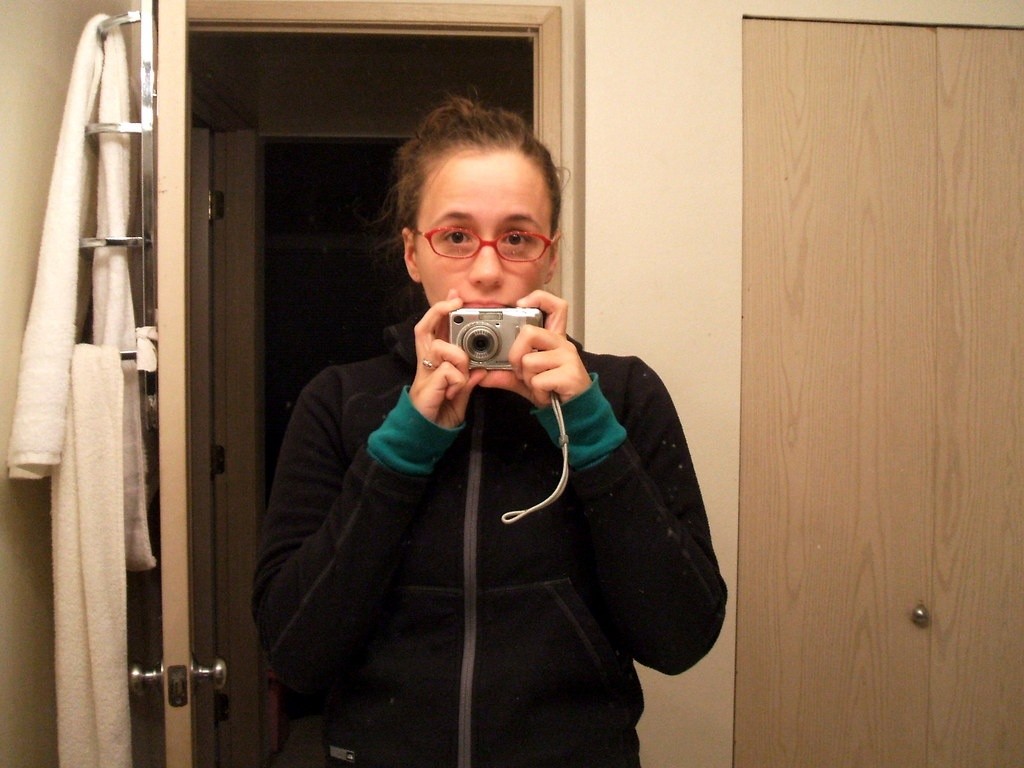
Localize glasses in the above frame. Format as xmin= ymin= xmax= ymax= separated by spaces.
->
xmin=412 ymin=226 xmax=560 ymax=263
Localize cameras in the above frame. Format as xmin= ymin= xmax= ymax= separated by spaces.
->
xmin=448 ymin=308 xmax=544 ymax=371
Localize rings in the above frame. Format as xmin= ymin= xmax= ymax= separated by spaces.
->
xmin=423 ymin=360 xmax=434 ymax=370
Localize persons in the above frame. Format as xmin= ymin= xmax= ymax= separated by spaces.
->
xmin=249 ymin=96 xmax=728 ymax=768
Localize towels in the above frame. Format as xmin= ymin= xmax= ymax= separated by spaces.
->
xmin=49 ymin=341 xmax=134 ymax=768
xmin=7 ymin=10 xmax=157 ymax=570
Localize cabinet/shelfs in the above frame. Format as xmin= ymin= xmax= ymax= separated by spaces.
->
xmin=733 ymin=17 xmax=1024 ymax=767
